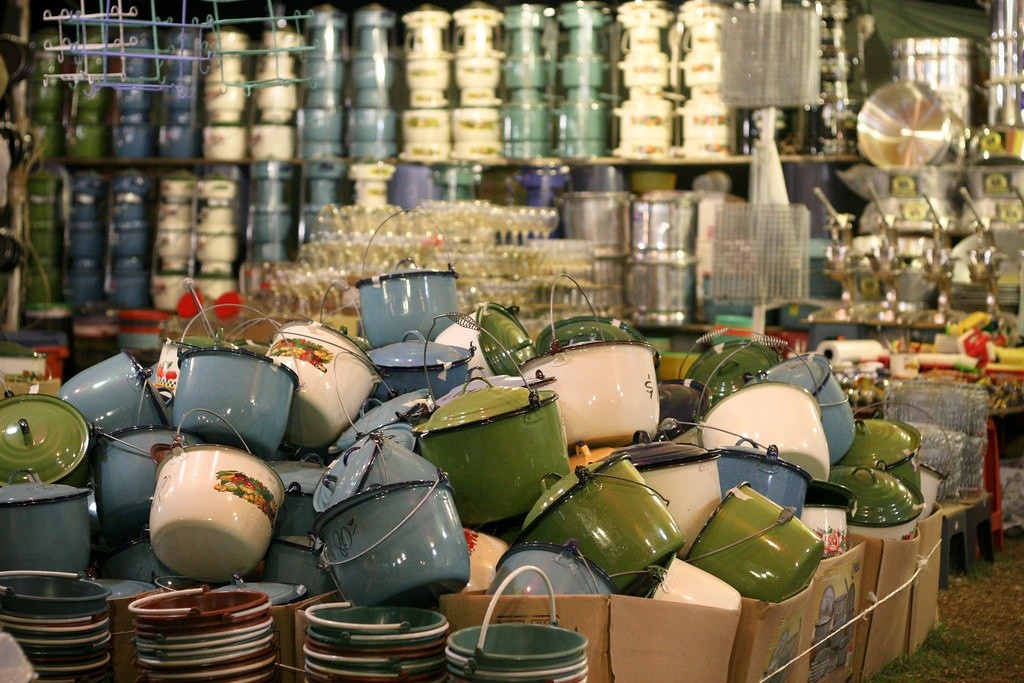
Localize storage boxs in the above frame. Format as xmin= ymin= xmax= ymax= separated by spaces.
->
xmin=105 ymin=506 xmax=953 ymax=683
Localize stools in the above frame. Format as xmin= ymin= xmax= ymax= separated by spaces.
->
xmin=943 ymin=417 xmax=1005 ymax=588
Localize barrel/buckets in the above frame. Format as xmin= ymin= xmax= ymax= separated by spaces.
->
xmin=0 ymin=207 xmax=954 ymax=683
